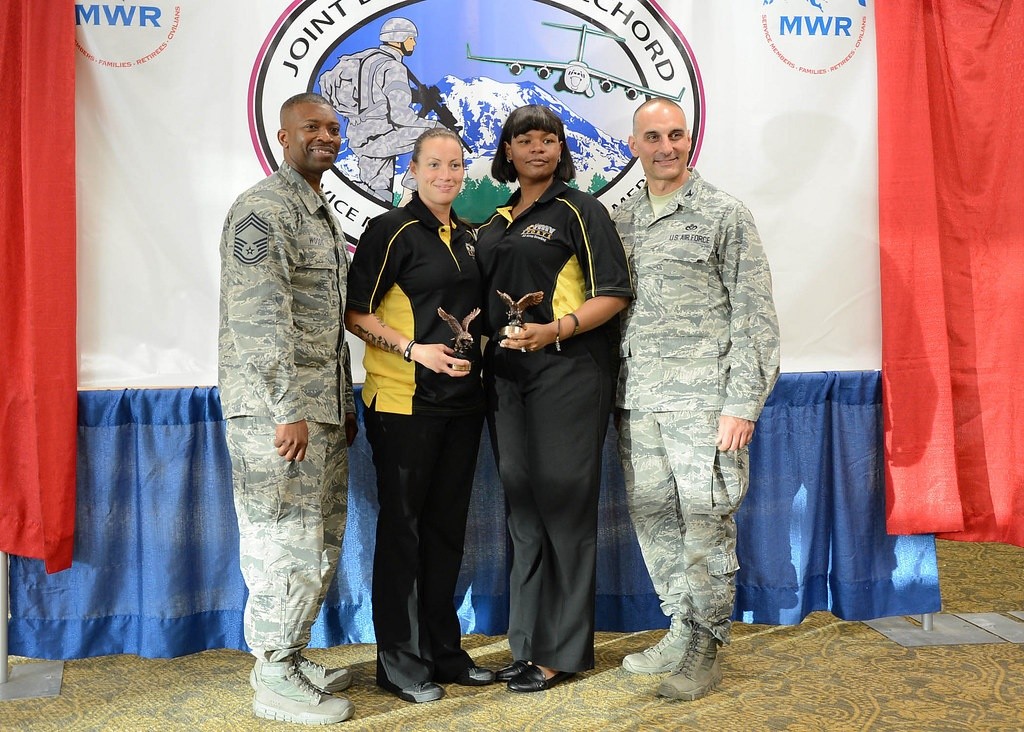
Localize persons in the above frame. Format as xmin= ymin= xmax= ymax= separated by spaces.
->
xmin=217 ymin=94 xmax=353 ymax=725
xmin=611 ymin=95 xmax=780 ymax=699
xmin=341 ymin=128 xmax=493 ymax=701
xmin=476 ymin=104 xmax=633 ymax=692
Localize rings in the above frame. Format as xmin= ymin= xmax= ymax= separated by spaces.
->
xmin=520 ymin=346 xmax=527 ymax=352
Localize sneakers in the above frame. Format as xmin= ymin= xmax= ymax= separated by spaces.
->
xmin=249 ymin=650 xmax=352 ymax=693
xmin=252 ymin=661 xmax=356 ymax=725
xmin=622 ymin=613 xmax=692 ymax=673
xmin=657 ymin=626 xmax=723 ymax=701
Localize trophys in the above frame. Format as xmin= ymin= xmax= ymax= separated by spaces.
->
xmin=496 ymin=288 xmax=544 ymax=342
xmin=437 ymin=307 xmax=481 ymax=372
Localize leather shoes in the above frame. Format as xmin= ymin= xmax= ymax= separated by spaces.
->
xmin=493 ymin=660 xmax=534 ymax=682
xmin=507 ymin=664 xmax=577 ymax=693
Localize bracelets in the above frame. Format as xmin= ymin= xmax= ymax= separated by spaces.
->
xmin=403 ymin=340 xmax=417 ymax=363
xmin=555 ymin=319 xmax=561 ymax=351
xmin=563 ymin=313 xmax=579 ymax=337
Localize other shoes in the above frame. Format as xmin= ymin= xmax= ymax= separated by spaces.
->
xmin=453 ymin=665 xmax=496 ymax=686
xmin=393 ymin=682 xmax=445 ymax=703
